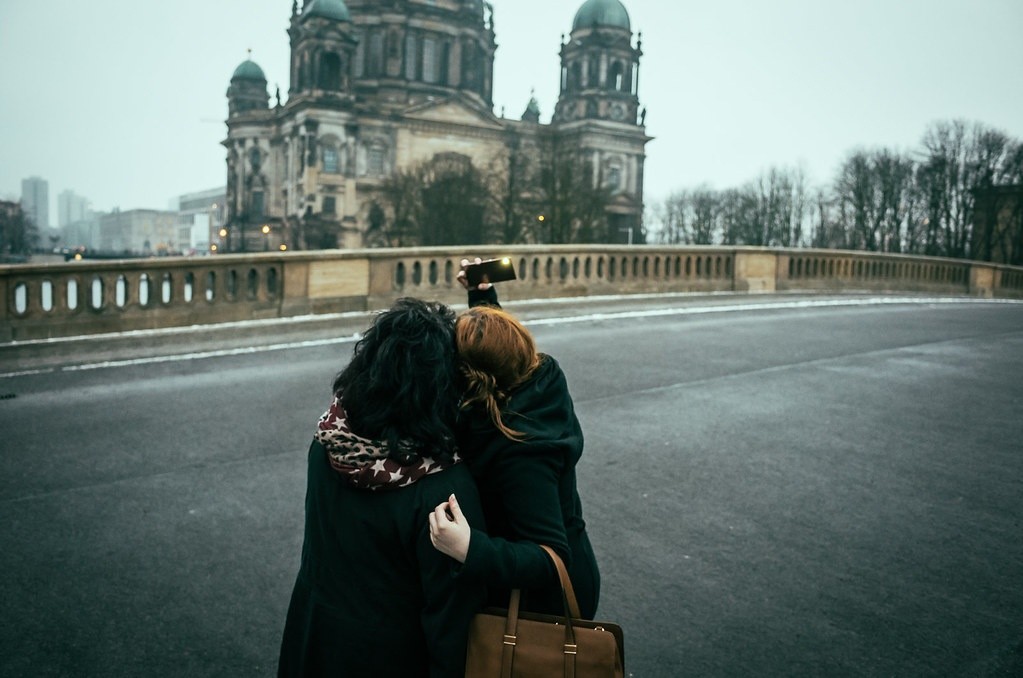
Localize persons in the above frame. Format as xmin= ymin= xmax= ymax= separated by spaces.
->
xmin=429 ymin=257 xmax=601 ymax=620
xmin=276 ymin=296 xmax=487 ymax=678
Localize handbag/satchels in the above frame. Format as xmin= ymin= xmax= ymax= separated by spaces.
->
xmin=465 ymin=543 xmax=624 ymax=678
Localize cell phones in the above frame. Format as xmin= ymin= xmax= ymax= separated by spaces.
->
xmin=464 ymin=259 xmax=517 ymax=288
